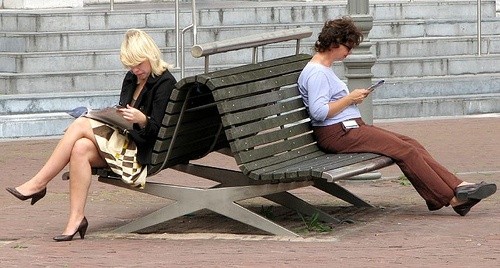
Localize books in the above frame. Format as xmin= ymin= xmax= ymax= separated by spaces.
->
xmin=62 ymin=103 xmax=134 ymax=132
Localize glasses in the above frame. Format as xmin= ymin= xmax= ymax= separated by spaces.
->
xmin=339 ymin=42 xmax=352 ymax=52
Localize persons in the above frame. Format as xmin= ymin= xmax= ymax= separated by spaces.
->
xmin=5 ymin=28 xmax=177 ymax=242
xmin=297 ymin=17 xmax=496 ymax=217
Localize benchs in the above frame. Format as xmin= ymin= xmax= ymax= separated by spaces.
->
xmin=62 ymin=26 xmax=395 ymax=238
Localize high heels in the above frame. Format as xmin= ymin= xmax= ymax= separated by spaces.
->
xmin=53 ymin=216 xmax=88 ymax=240
xmin=5 ymin=188 xmax=46 ymax=205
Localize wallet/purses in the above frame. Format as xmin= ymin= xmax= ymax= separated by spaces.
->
xmin=92 ymin=107 xmax=134 ymax=131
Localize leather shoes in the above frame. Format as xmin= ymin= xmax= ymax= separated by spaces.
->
xmin=452 ymin=198 xmax=481 ymax=216
xmin=455 ymin=181 xmax=497 ymax=200
